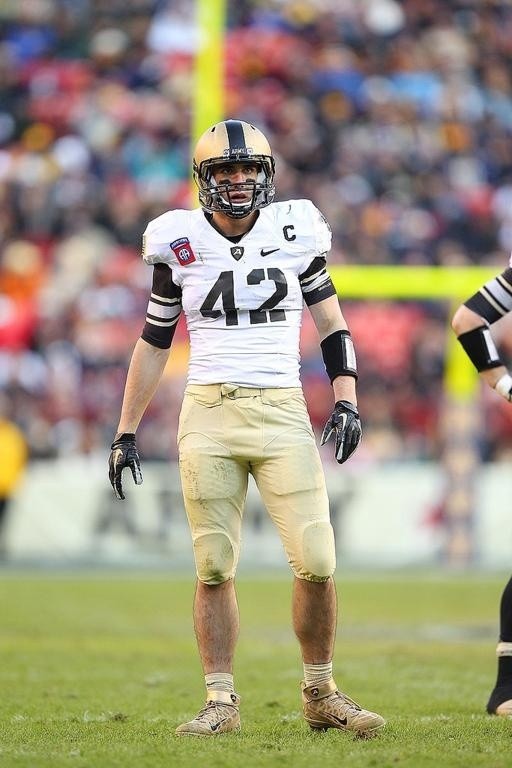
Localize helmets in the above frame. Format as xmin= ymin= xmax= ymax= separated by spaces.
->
xmin=194 ymin=118 xmax=277 ymax=215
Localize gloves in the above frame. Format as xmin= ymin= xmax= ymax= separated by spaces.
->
xmin=107 ymin=433 xmax=145 ymax=501
xmin=320 ymin=401 xmax=364 ymax=462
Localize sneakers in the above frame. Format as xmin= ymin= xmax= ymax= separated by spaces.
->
xmin=299 ymin=677 xmax=387 ymax=733
xmin=174 ymin=690 xmax=241 ymax=736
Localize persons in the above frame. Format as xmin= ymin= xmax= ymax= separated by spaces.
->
xmin=452 ymin=264 xmax=511 ymax=718
xmin=108 ymin=116 xmax=388 ymax=735
